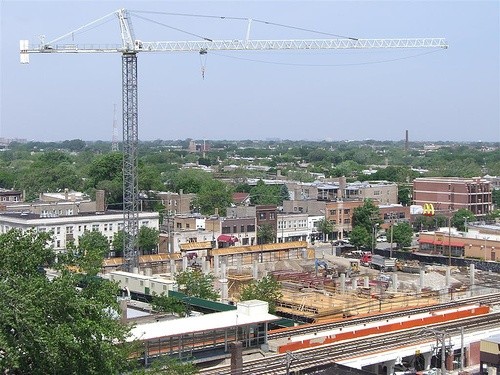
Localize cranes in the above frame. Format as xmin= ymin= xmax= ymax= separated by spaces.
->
xmin=18 ymin=8 xmax=447 ymax=276
